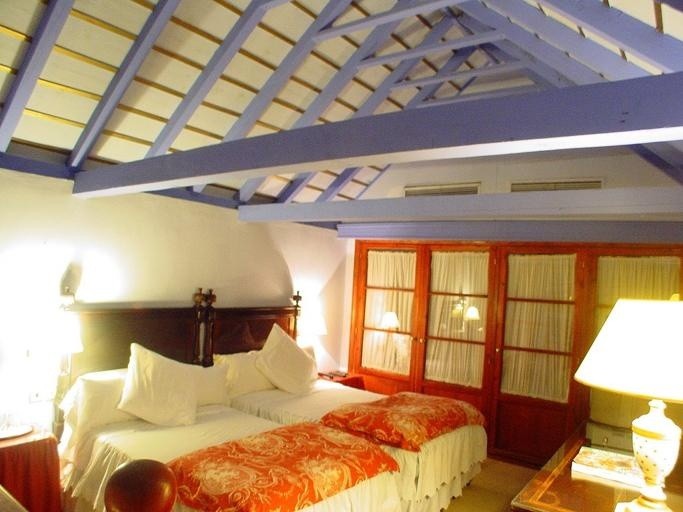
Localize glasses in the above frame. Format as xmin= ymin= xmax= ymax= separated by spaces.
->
xmin=572 ymin=296 xmax=683 ymax=512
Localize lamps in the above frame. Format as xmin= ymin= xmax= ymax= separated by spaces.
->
xmin=572 ymin=296 xmax=683 ymax=512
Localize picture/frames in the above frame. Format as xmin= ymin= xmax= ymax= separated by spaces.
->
xmin=0 ymin=433 xmax=62 ymax=511
xmin=506 ymin=412 xmax=682 ymax=512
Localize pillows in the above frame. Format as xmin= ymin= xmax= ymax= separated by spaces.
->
xmin=119 ymin=344 xmax=202 ymax=428
xmin=254 ymin=324 xmax=316 ymax=395
xmin=200 ymin=364 xmax=231 ymax=407
xmin=214 ymin=351 xmax=277 ymax=395
xmin=77 ymin=378 xmax=136 ymax=427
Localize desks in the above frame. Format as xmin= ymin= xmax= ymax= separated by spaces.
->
xmin=0 ymin=433 xmax=62 ymax=511
xmin=506 ymin=412 xmax=682 ymax=512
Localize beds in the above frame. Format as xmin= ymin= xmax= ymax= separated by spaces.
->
xmin=204 ymin=303 xmax=489 ymax=508
xmin=48 ymin=303 xmax=399 ymax=511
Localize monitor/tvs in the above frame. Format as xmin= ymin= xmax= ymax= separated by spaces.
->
xmin=572 ymin=446 xmax=660 ymax=489
